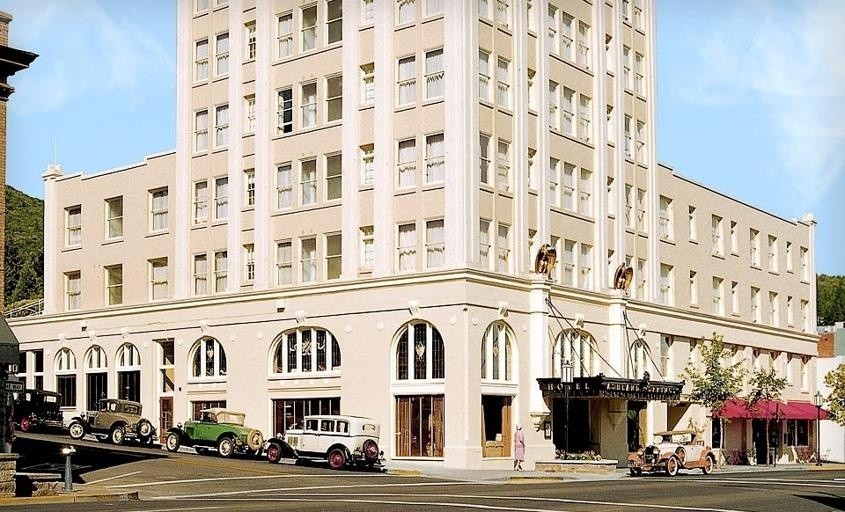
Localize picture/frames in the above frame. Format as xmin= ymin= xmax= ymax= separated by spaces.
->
xmin=544 ymin=421 xmax=551 ymax=440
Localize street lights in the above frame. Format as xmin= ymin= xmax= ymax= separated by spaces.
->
xmin=561 ymin=360 xmax=574 ymax=460
xmin=814 ymin=390 xmax=823 ymax=466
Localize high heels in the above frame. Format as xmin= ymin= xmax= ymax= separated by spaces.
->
xmin=514 ymin=468 xmax=523 ymax=471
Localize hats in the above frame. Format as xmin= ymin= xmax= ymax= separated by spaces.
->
xmin=516 ymin=423 xmax=522 ymax=430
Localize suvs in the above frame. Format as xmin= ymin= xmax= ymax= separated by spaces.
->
xmin=627 ymin=430 xmax=718 ymax=476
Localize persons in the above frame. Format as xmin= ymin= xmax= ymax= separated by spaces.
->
xmin=617 ymin=261 xmax=630 ymax=289
xmin=535 ymin=244 xmax=551 ymax=274
xmin=514 ymin=422 xmax=525 ymax=471
